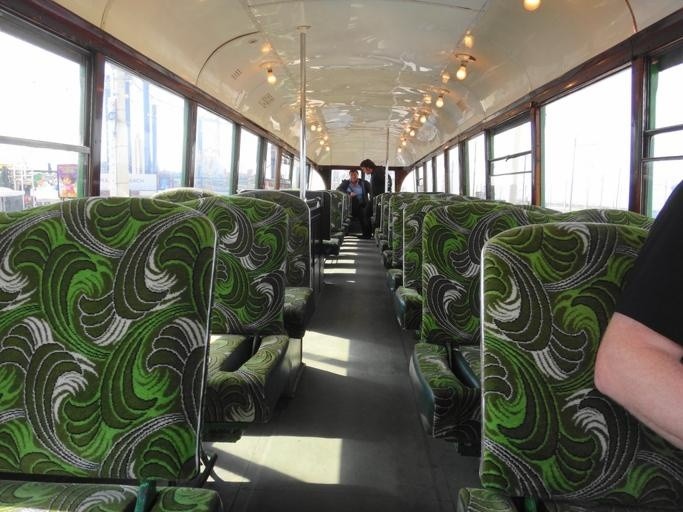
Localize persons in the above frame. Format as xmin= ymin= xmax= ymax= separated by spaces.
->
xmin=335 ymin=167 xmax=373 ymax=235
xmin=590 ymin=179 xmax=682 ymax=454
xmin=355 ymin=158 xmax=393 ymax=239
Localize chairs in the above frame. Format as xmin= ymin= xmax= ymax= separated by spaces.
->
xmin=372 ymin=184 xmax=660 ymax=451
xmin=1 ymin=194 xmax=349 ymax=441
xmin=456 ymin=221 xmax=681 ymax=510
xmin=0 ymin=197 xmax=224 ymax=510
